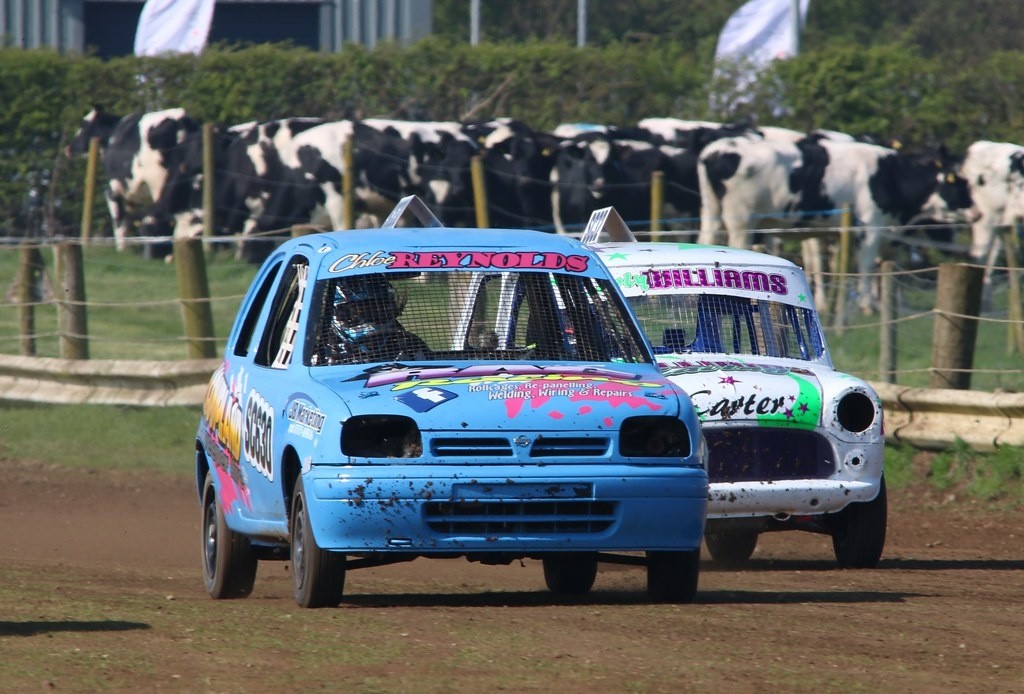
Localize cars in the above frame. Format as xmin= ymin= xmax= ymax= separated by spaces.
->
xmin=453 ymin=202 xmax=890 ymax=571
xmin=192 ymin=194 xmax=712 ymax=612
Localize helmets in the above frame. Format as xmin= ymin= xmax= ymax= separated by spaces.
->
xmin=332 ymin=273 xmax=399 ymax=340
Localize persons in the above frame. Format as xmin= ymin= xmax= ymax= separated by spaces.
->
xmin=310 ymin=275 xmax=433 ymax=369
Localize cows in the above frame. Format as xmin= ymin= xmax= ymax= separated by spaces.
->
xmin=68 ymin=104 xmax=1023 ymax=322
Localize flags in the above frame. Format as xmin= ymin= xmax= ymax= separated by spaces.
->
xmin=133 ymin=0 xmax=218 ymax=81
xmin=710 ymin=0 xmax=812 ymax=125
